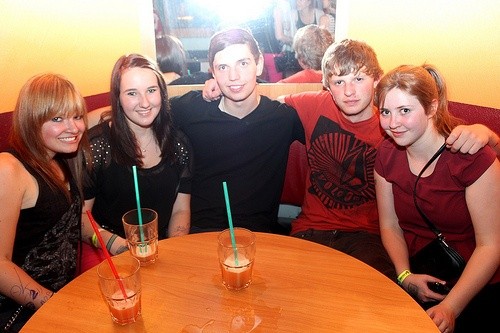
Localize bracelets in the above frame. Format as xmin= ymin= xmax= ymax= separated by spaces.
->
xmin=106 ymin=234 xmax=118 ymax=252
xmin=92 ymin=228 xmax=106 ymax=248
xmin=396 ymin=269 xmax=412 ymax=285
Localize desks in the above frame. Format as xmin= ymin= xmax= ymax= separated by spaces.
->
xmin=19 ymin=232 xmax=441 ymax=333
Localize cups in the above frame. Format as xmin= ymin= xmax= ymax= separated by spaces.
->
xmin=122 ymin=208 xmax=159 ymax=267
xmin=97 ymin=254 xmax=142 ymax=324
xmin=217 ymin=227 xmax=256 ymax=290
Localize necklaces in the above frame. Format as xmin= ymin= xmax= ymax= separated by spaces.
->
xmin=134 ymin=136 xmax=153 ymax=152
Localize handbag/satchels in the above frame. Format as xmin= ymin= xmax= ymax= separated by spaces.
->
xmin=409 ymin=238 xmax=468 ymax=301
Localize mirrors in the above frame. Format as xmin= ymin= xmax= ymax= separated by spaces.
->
xmin=152 ymin=0 xmax=336 ymax=88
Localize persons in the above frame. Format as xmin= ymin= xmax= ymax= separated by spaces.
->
xmin=78 ymin=53 xmax=194 ymax=256
xmin=273 ymin=0 xmax=334 ymax=82
xmin=155 ymin=34 xmax=188 ymax=85
xmin=0 ymin=72 xmax=131 ymax=333
xmin=164 ymin=28 xmax=308 ymax=238
xmin=374 ymin=63 xmax=500 ymax=333
xmin=202 ymin=38 xmax=500 ymax=271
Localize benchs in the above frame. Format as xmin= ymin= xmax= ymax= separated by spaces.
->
xmin=0 ymin=92 xmax=500 ymax=225
